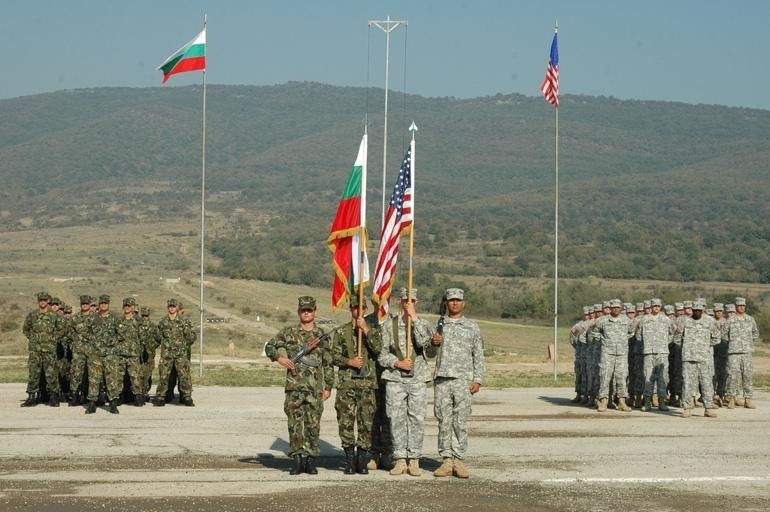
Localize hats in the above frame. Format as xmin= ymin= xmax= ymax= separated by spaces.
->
xmin=167 ymin=299 xmax=183 ymax=310
xmin=122 ymin=298 xmax=150 ymax=316
xmin=38 ymin=291 xmax=72 ymax=313
xmin=664 ymin=298 xmax=706 ymax=316
xmin=445 ymin=288 xmax=464 ymax=301
xmin=349 ymin=295 xmax=367 ymax=307
xmin=623 ymin=299 xmax=662 ymax=313
xmin=81 ymin=294 xmax=110 ymax=305
xmin=708 ymin=297 xmax=746 ymax=316
xmin=400 ymin=286 xmax=417 ymax=300
xmin=582 ymin=299 xmax=621 ymax=314
xmin=298 ymin=296 xmax=316 ymax=311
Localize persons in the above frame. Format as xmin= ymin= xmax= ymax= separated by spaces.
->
xmin=332 ymin=294 xmax=383 ymax=474
xmin=264 ymin=297 xmax=334 ymax=477
xmin=567 ymin=297 xmax=759 ymax=419
xmin=427 ymin=287 xmax=487 ymax=478
xmin=363 ymin=297 xmax=396 ymax=471
xmin=21 ymin=292 xmax=197 ymax=414
xmin=378 ymin=286 xmax=432 ymax=476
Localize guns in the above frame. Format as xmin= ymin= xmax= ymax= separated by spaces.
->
xmin=289 ymin=324 xmax=344 ymax=377
xmin=436 ymin=293 xmax=446 ymax=334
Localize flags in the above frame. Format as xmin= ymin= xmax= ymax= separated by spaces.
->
xmin=540 ymin=32 xmax=560 ymax=109
xmin=327 ymin=135 xmax=370 ymax=312
xmin=156 ymin=29 xmax=206 ymax=85
xmin=370 ymin=143 xmax=415 ymax=325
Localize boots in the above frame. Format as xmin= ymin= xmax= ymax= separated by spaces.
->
xmin=181 ymin=396 xmax=194 ymax=407
xmin=632 ymin=393 xmax=670 ymax=411
xmin=134 ymin=393 xmax=149 ymax=407
xmin=433 ymin=456 xmax=469 ymax=477
xmin=713 ymin=394 xmax=757 ymax=409
xmin=22 ymin=390 xmax=47 ymax=406
xmin=345 ymin=446 xmax=421 ymax=477
xmin=290 ymin=454 xmax=317 ymax=474
xmin=155 ymin=391 xmax=174 ymax=406
xmin=48 ymin=392 xmax=85 ymax=406
xmin=86 ymin=401 xmax=96 ymax=413
xmin=668 ymin=393 xmax=702 ymax=407
xmin=704 ymin=407 xmax=716 ymax=417
xmin=110 ymin=398 xmax=118 ymax=414
xmin=572 ymin=397 xmax=632 ymax=412
xmin=681 ymin=408 xmax=691 ymax=418
